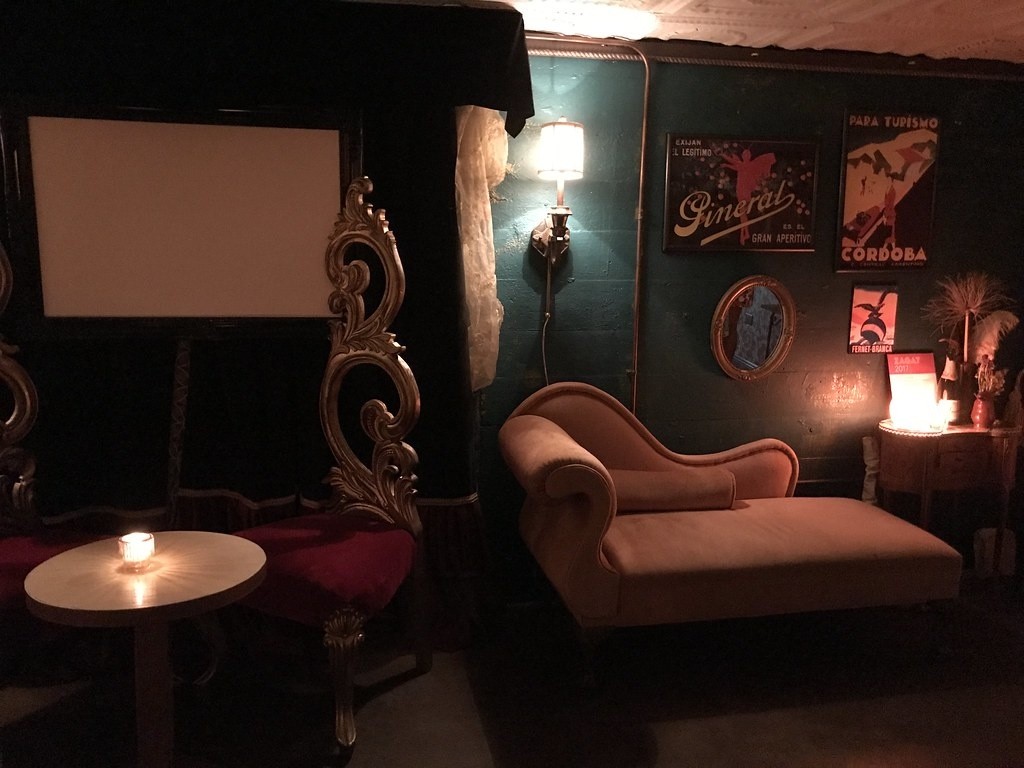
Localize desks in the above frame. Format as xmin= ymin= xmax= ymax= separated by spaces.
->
xmin=24 ymin=530 xmax=268 ymax=768
xmin=878 ymin=419 xmax=1021 ymax=582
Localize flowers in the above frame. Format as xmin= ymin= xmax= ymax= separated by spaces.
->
xmin=975 ymin=354 xmax=1009 ymax=398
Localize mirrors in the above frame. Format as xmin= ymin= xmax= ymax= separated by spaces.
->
xmin=710 ymin=275 xmax=797 ymax=381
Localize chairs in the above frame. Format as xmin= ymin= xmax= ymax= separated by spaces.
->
xmin=192 ymin=175 xmax=429 ymax=757
xmin=1 ymin=242 xmax=136 ymax=602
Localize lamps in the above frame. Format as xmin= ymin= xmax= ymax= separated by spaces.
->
xmin=532 ymin=117 xmax=584 ymax=267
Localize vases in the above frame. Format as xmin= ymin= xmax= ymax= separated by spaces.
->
xmin=971 ymin=398 xmax=995 ymax=430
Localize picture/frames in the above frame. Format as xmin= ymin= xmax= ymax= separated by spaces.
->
xmin=848 ymin=285 xmax=899 ymax=353
xmin=662 ymin=133 xmax=824 ymax=255
xmin=836 ymin=107 xmax=945 ymax=273
xmin=886 ymin=352 xmax=939 ymax=397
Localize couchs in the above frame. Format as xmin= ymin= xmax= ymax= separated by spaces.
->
xmin=498 ymin=381 xmax=964 ymax=689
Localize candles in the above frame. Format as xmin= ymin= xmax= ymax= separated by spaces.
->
xmin=118 ymin=531 xmax=156 ymax=568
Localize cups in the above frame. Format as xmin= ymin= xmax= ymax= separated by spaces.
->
xmin=119 ymin=533 xmax=155 ymax=569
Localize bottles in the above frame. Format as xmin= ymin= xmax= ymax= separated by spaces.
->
xmin=935 ymin=347 xmax=960 ymax=426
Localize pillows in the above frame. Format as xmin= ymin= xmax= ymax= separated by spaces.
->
xmin=608 ymin=468 xmax=736 ymax=513
